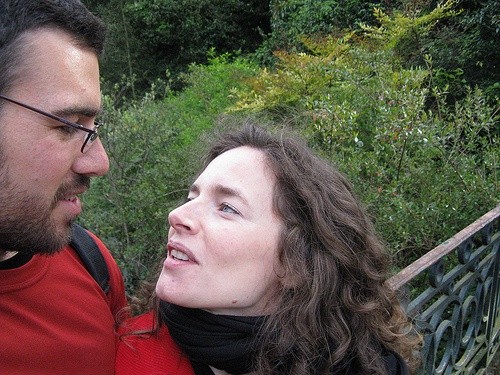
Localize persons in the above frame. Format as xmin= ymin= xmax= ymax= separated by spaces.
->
xmin=110 ymin=119 xmax=423 ymax=375
xmin=0 ymin=1 xmax=131 ymax=375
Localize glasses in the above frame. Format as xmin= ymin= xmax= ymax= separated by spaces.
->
xmin=1 ymin=95 xmax=105 ymax=154
